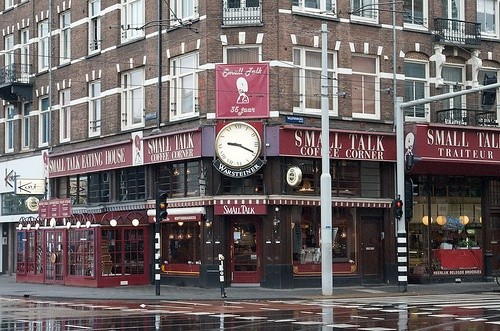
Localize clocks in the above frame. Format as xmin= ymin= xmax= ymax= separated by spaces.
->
xmin=214 ymin=121 xmax=262 ymax=170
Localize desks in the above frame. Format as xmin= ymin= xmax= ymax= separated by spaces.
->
xmin=430 ymin=247 xmax=484 ymax=275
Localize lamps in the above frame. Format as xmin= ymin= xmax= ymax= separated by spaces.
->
xmin=459 ymin=216 xmax=469 ymax=225
xmin=422 ymin=215 xmax=433 ymax=225
xmin=436 ymin=215 xmax=447 ymax=225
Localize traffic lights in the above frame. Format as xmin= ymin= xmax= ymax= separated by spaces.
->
xmin=394 ymin=198 xmax=403 ymax=220
xmin=405 ymin=177 xmax=418 ymax=223
xmin=156 ymin=191 xmax=168 ymax=222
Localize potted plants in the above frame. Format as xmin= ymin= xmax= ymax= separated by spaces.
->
xmin=456 ymin=241 xmax=468 ymax=249
xmin=468 ymin=240 xmax=480 ymax=249
